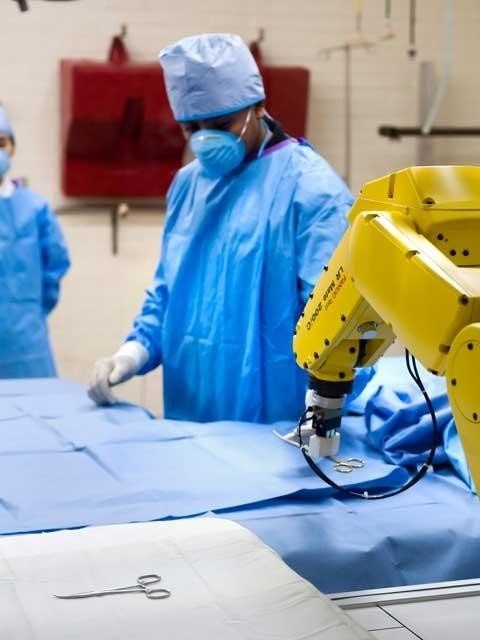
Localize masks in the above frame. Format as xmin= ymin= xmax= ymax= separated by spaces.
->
xmin=0 ymin=149 xmax=11 ymax=176
xmin=187 ymin=108 xmax=253 ymax=181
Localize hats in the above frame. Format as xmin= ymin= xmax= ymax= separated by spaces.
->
xmin=0 ymin=102 xmax=14 ymax=137
xmin=157 ymin=32 xmax=266 ymax=123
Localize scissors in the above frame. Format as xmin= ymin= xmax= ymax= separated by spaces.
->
xmin=326 ymin=456 xmax=364 ymax=473
xmin=53 ymin=574 xmax=171 ymax=599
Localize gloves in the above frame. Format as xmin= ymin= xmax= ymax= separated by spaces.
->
xmin=85 ymin=339 xmax=151 ymax=406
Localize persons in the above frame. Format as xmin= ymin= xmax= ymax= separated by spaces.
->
xmin=84 ymin=30 xmax=388 ymax=428
xmin=0 ymin=105 xmax=71 ymax=378
